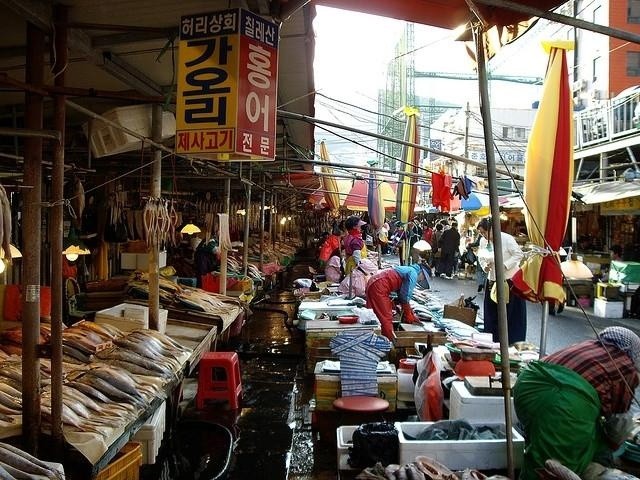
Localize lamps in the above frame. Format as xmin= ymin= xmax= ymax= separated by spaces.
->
xmin=60 ymin=220 xmax=92 ymax=263
xmin=178 ymin=220 xmax=203 ymax=235
xmin=411 ymin=183 xmax=432 ymax=254
xmin=0 ymin=241 xmax=22 ymax=275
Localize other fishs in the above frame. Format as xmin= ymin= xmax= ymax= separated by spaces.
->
xmin=0 ymin=441 xmax=65 ymax=480
xmin=434 ymin=317 xmax=480 ymax=341
xmin=225 ymin=255 xmax=263 ymax=281
xmin=0 ymin=321 xmax=194 ymax=437
xmin=127 ymin=270 xmax=242 ymax=315
xmin=385 ymin=463 xmax=511 ymax=480
xmin=396 ymin=288 xmax=449 ymax=320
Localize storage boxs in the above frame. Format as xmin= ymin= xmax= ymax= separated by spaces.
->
xmin=299 ymin=284 xmax=556 ymax=480
xmin=126 ymin=398 xmax=168 ymax=466
xmin=80 ymin=99 xmax=178 ymax=161
xmin=576 ymin=248 xmax=640 ymax=321
xmin=118 ymin=249 xmax=170 ymax=272
xmin=94 ymin=300 xmax=170 ymax=336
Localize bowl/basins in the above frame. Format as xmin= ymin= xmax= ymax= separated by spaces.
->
xmin=398 ymin=359 xmax=416 ymax=369
xmin=455 ymin=360 xmax=496 ymax=379
xmin=336 ymin=315 xmax=359 ymax=324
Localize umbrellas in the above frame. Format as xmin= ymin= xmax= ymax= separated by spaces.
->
xmin=367 ymin=160 xmax=384 ymax=269
xmin=395 ymin=107 xmax=420 ymax=265
xmin=427 ymin=192 xmax=491 ymax=218
xmin=309 ymin=172 xmax=399 ymax=212
xmin=320 ymin=141 xmax=340 ymax=218
xmin=510 ymin=40 xmax=575 ymax=361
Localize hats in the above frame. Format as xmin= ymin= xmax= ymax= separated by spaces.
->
xmin=417 ymin=265 xmax=431 ymax=289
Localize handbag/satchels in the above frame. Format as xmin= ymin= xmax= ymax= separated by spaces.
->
xmin=489 ymin=281 xmax=509 ymax=304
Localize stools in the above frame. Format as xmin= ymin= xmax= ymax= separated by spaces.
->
xmin=195 ymin=349 xmax=243 ymax=413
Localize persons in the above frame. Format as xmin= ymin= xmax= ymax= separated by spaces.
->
xmin=578 ymin=237 xmax=595 ymax=250
xmin=513 ymin=326 xmax=640 ymax=480
xmin=598 ymin=244 xmax=622 ymax=282
xmin=476 ymin=218 xmax=526 ymax=344
xmin=365 ymin=260 xmax=431 ymax=340
xmin=317 ymin=215 xmax=488 ymax=299
